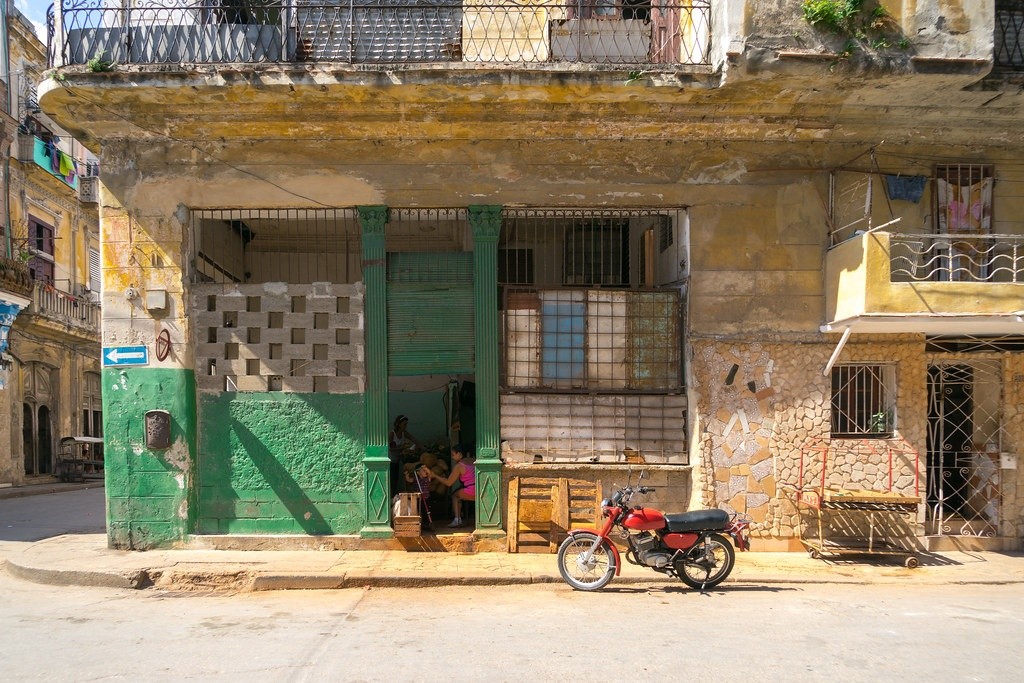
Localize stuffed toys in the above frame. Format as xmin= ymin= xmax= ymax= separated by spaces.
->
xmin=420 ymin=452 xmax=449 ymax=494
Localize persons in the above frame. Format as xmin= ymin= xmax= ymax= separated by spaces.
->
xmin=427 ymin=444 xmax=475 ymax=527
xmin=389 ymin=415 xmax=420 ymax=498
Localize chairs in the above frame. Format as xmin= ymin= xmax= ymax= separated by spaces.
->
xmin=457 ymin=500 xmax=475 ymax=529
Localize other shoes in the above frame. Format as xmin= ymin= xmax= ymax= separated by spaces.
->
xmin=447 ymin=519 xmax=462 ymax=527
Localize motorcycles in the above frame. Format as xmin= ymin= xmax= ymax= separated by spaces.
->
xmin=557 ymin=465 xmax=754 ymax=595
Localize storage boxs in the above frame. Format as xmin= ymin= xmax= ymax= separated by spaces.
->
xmin=394 ymin=492 xmax=422 ymax=538
